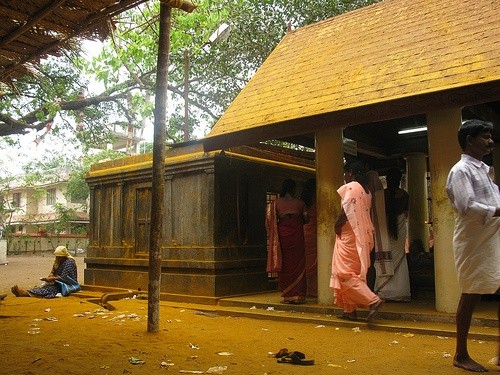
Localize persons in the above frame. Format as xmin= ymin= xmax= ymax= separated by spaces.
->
xmin=406 ymin=239 xmax=435 ymax=293
xmin=265 ymin=178 xmax=309 ymax=304
xmin=297 ymin=180 xmax=318 ymax=299
xmin=329 ymin=160 xmax=384 ymax=322
xmin=445 ymin=118 xmax=500 ymax=373
xmin=361 ymin=168 xmax=384 ymax=194
xmin=11 ymin=245 xmax=78 ymax=299
xmin=371 ymin=165 xmax=412 ymax=301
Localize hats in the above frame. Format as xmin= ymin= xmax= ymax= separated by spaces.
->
xmin=54 ymin=246 xmax=69 ymax=256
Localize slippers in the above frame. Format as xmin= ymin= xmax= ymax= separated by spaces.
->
xmin=336 ymin=314 xmax=357 ymax=321
xmin=275 ymin=348 xmax=306 ymax=359
xmin=366 ymin=299 xmax=385 ymax=322
xmin=277 ymin=351 xmax=315 ymax=365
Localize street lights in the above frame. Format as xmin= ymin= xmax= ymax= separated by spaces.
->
xmin=184 ymin=23 xmax=230 ymax=142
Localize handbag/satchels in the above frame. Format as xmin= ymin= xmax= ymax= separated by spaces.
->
xmin=55 ymin=274 xmax=81 ymax=297
